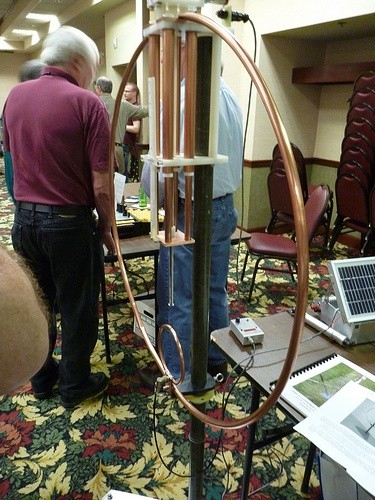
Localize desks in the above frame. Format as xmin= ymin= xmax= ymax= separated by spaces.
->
xmin=91 ymin=183 xmax=252 ymax=364
xmin=210 ymin=305 xmax=375 ymax=500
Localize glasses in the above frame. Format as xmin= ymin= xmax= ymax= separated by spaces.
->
xmin=123 ymin=90 xmax=135 ymax=92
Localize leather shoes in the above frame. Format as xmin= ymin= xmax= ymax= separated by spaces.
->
xmin=207 ymin=362 xmax=227 ymax=383
xmin=140 ymin=367 xmax=163 ymax=388
xmin=61 ymin=372 xmax=108 ymax=409
xmin=31 ymin=357 xmax=60 ymax=400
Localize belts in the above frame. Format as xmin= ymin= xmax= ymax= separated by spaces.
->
xmin=14 ymin=201 xmax=92 ymax=215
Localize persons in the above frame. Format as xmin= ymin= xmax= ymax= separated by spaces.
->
xmin=95 ymin=78 xmax=150 ymax=175
xmin=3 ymin=58 xmax=44 ymax=209
xmin=3 ymin=26 xmax=117 ymax=407
xmin=140 ymin=60 xmax=244 ymax=394
xmin=123 ymin=83 xmax=141 ymax=182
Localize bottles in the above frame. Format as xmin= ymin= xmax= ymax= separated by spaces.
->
xmin=139 ymin=183 xmax=147 ymax=207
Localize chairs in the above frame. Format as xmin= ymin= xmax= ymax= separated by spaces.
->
xmin=240 ymin=183 xmax=331 ymax=303
xmin=265 ymin=70 xmax=375 ymax=257
